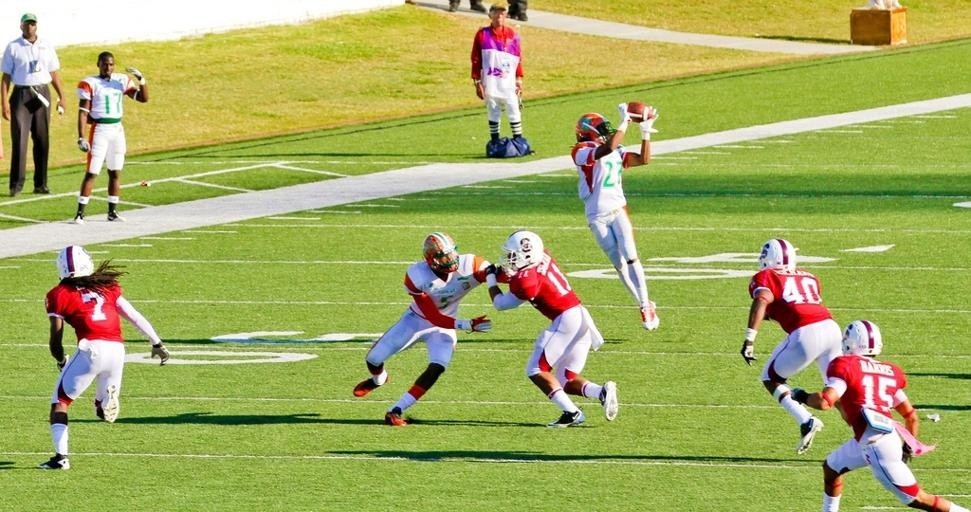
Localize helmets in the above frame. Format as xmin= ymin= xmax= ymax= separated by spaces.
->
xmin=758 ymin=238 xmax=799 ymax=269
xmin=56 ymin=245 xmax=94 ymax=279
xmin=500 ymin=229 xmax=544 ymax=274
xmin=841 ymin=319 xmax=884 ymax=356
xmin=575 ymin=112 xmax=616 ymax=144
xmin=422 ymin=230 xmax=460 ymax=273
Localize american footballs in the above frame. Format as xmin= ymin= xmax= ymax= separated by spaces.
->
xmin=627 ymin=103 xmax=653 ymax=122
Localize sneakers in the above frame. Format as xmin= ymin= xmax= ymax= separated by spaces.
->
xmin=32 ymin=188 xmax=52 ymax=194
xmin=10 ymin=189 xmax=20 ymax=197
xmin=106 ymin=211 xmax=128 ymax=222
xmin=38 ymin=452 xmax=70 ymax=470
xmin=353 ymin=373 xmax=389 ymax=397
xmin=103 ymin=383 xmax=119 ymax=423
xmin=599 ymin=381 xmax=619 ymax=422
xmin=74 ymin=214 xmax=83 ymax=225
xmin=640 ymin=301 xmax=660 ymax=331
xmin=385 ymin=411 xmax=406 ymax=427
xmin=797 ymin=415 xmax=823 ymax=455
xmin=548 ymin=410 xmax=585 ymax=428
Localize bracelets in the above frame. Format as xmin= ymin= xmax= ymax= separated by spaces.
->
xmin=139 ymin=76 xmax=145 ymax=86
xmin=474 ymin=80 xmax=481 ymax=85
xmin=517 ymin=80 xmax=523 ymax=84
xmin=486 ymin=273 xmax=498 ymax=288
xmin=745 ymin=327 xmax=757 ymax=343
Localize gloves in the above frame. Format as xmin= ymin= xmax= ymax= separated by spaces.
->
xmin=484 ymin=263 xmax=497 ymax=277
xmin=77 ymin=138 xmax=91 ymax=153
xmin=640 ymin=105 xmax=659 ymax=140
xmin=617 ymin=102 xmax=630 ymax=134
xmin=901 ymin=442 xmax=913 ymax=464
xmin=57 ymin=353 xmax=69 ymax=371
xmin=740 ymin=327 xmax=758 ymax=366
xmin=151 ymin=341 xmax=171 ymax=366
xmin=126 ymin=67 xmax=145 ymax=83
xmin=471 ymin=314 xmax=492 ymax=334
xmin=790 ymin=387 xmax=808 ymax=402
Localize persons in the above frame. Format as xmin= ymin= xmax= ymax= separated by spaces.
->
xmin=38 ymin=244 xmax=171 ymax=472
xmin=507 ymin=0 xmax=528 ymax=22
xmin=787 ymin=319 xmax=971 ymax=511
xmin=568 ymin=101 xmax=662 ymax=334
xmin=469 ymin=3 xmax=525 ymax=142
xmin=448 ymin=0 xmax=486 ymax=16
xmin=73 ymin=50 xmax=148 ymax=224
xmin=739 ymin=236 xmax=848 ymax=457
xmin=476 ymin=228 xmax=619 ymax=427
xmin=0 ymin=11 xmax=66 ymax=198
xmin=351 ymin=229 xmax=506 ymax=425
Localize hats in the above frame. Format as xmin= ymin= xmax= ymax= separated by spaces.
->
xmin=488 ymin=0 xmax=508 ymax=11
xmin=20 ymin=13 xmax=37 ymax=24
